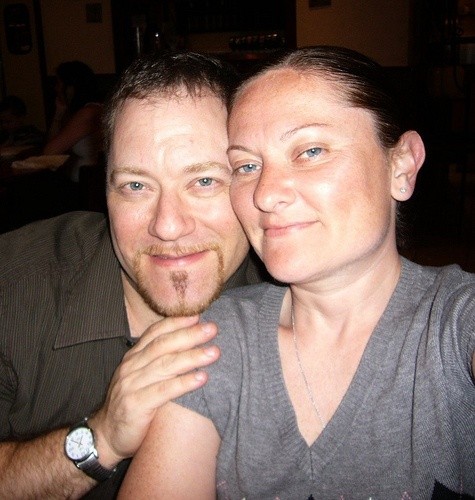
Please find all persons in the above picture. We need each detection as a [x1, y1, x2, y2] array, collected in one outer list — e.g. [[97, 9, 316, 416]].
[[114, 41, 474, 500], [2, 52, 273, 499], [0, 96, 47, 149], [41, 59, 112, 184]]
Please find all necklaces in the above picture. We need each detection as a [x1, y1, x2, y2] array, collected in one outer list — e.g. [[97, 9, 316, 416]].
[[287, 298, 331, 433]]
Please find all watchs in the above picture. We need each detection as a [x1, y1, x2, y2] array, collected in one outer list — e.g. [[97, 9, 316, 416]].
[[64, 411, 117, 484]]
